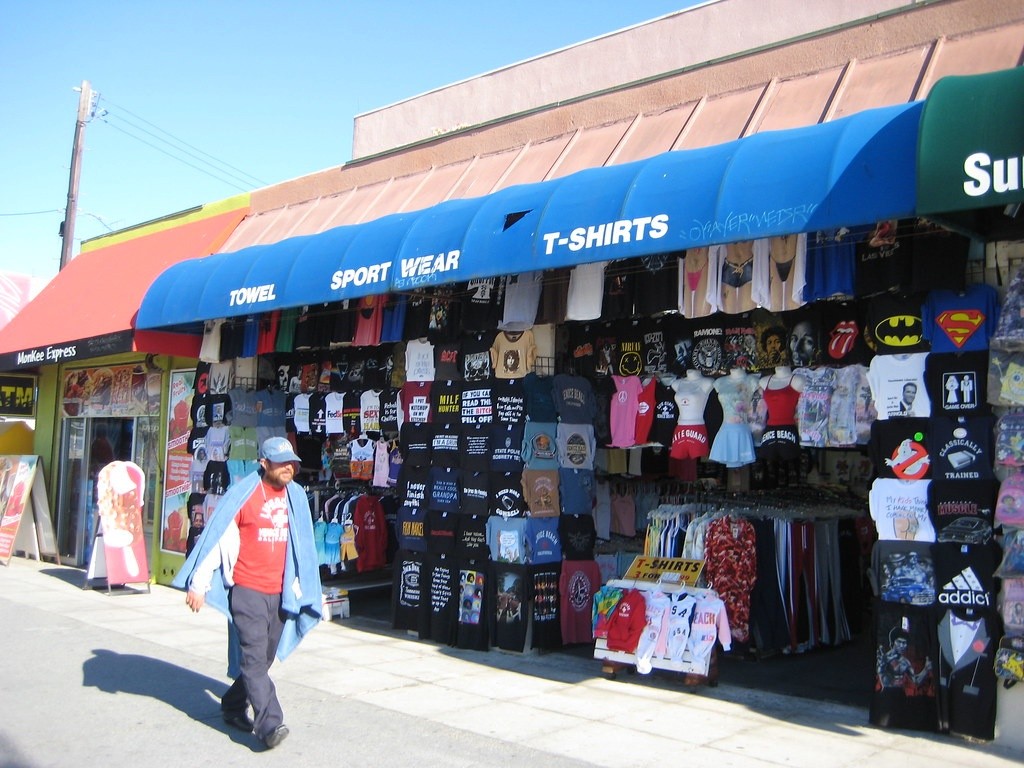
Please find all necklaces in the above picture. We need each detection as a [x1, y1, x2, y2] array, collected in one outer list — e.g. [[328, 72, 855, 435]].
[[259, 475, 288, 532]]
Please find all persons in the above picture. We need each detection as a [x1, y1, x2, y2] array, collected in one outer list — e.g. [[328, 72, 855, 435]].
[[682, 229, 824, 319], [900, 382, 919, 415], [670, 363, 806, 470], [185, 436, 332, 750], [192, 512, 204, 529], [876, 626, 932, 699]]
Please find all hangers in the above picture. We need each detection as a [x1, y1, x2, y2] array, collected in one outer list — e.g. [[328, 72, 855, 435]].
[[616, 477, 868, 525]]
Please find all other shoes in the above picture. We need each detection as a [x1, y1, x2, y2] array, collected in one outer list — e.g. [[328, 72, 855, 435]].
[[264, 722, 290, 748], [223, 709, 255, 731]]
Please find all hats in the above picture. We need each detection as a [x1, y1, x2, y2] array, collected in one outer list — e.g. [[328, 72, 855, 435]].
[[259, 436, 302, 463]]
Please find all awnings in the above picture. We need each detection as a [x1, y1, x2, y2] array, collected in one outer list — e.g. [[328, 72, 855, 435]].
[[133, 98, 932, 335], [0, 205, 251, 372], [916, 64, 1024, 245]]
